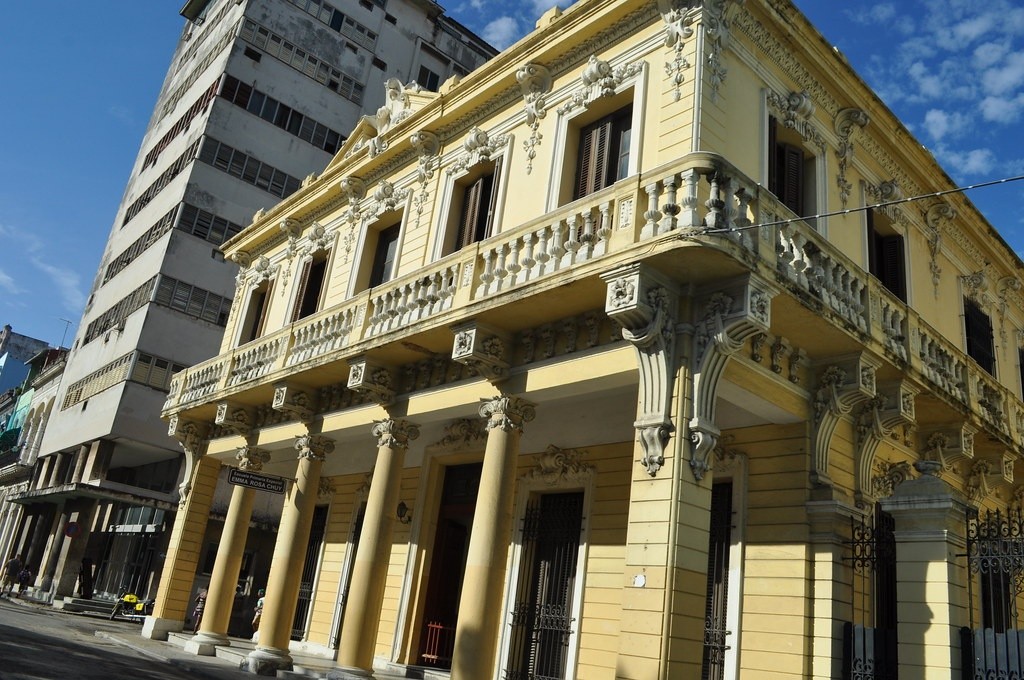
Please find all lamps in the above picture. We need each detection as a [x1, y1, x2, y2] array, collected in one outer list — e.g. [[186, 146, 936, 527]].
[[396, 501, 412, 525]]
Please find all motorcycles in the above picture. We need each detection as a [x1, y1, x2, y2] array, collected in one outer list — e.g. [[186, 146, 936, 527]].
[[110, 585, 154, 623]]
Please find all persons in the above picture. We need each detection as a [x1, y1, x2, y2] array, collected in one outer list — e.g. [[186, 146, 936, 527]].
[[251, 589, 265, 631], [15, 565, 33, 598], [0, 554, 23, 597], [193, 585, 210, 633]]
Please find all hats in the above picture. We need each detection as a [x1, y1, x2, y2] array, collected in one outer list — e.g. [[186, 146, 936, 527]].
[[259, 589, 265, 593]]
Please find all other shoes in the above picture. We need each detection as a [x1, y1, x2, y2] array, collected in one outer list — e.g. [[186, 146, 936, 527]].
[[15, 594, 18, 598], [0, 592, 3, 597], [7, 594, 12, 597]]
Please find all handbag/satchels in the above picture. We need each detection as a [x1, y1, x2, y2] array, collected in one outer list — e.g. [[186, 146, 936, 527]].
[[193, 611, 197, 616]]
